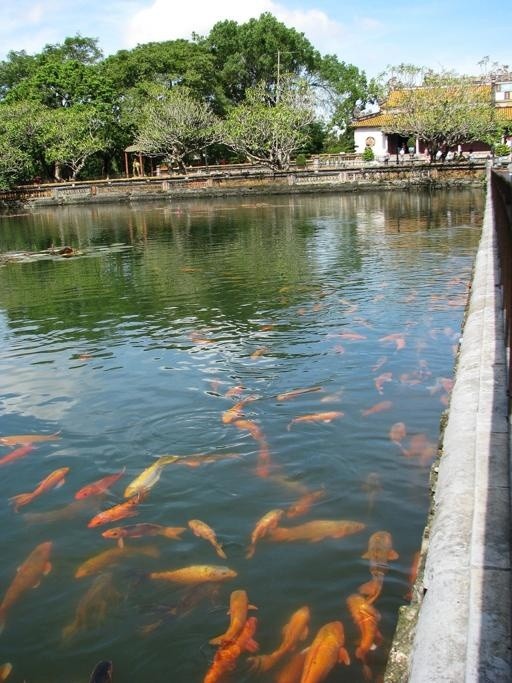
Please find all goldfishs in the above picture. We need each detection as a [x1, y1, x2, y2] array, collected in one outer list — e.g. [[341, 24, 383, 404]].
[[1, 265, 480, 682]]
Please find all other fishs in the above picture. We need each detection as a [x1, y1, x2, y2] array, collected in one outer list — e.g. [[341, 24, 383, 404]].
[[1, 265, 480, 682]]
